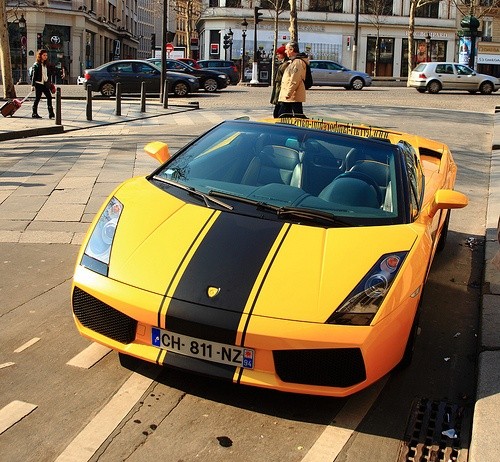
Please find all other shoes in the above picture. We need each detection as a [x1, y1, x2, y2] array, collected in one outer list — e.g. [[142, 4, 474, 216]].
[[32, 113, 42, 118], [49, 114, 56, 120]]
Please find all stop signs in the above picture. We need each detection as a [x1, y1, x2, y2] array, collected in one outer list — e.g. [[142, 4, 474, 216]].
[[167, 44, 174, 52]]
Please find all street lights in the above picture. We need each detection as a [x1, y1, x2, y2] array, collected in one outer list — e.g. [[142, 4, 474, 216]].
[[227, 30, 234, 60], [425, 36, 432, 62], [18, 17, 32, 86], [241, 20, 248, 82]]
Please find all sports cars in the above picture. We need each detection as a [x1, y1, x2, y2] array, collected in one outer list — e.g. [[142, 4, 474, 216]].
[[71, 115, 470, 396]]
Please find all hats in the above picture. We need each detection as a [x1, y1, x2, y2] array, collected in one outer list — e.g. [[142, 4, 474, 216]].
[[276, 46, 287, 57]]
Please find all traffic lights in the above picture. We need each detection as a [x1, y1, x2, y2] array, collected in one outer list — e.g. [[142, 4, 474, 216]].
[[223, 36, 230, 50], [254, 6, 263, 23], [166, 31, 176, 43]]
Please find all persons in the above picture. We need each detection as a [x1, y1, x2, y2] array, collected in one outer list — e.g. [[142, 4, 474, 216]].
[[31, 49, 63, 119], [270, 40, 309, 118], [415, 42, 431, 62], [303, 44, 313, 60], [245, 50, 250, 61], [257, 43, 266, 60], [461, 42, 470, 52]]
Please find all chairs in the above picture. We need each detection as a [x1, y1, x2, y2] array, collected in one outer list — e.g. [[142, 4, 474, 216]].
[[241, 132, 390, 208], [447, 66, 452, 71]]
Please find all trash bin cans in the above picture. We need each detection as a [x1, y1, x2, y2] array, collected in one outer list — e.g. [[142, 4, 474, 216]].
[[259, 62, 270, 82]]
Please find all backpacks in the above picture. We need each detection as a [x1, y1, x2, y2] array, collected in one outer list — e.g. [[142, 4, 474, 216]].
[[292, 57, 313, 90]]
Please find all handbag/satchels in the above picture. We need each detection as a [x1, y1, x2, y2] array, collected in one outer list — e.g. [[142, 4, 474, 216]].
[[50, 84, 56, 94]]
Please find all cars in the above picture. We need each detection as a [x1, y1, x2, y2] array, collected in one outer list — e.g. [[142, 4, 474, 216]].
[[83, 59, 198, 98], [147, 59, 229, 93], [309, 60, 372, 91], [407, 64, 500, 95], [186, 60, 240, 86], [175, 58, 201, 69]]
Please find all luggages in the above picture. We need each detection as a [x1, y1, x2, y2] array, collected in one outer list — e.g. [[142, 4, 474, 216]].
[[0, 91, 34, 117]]
